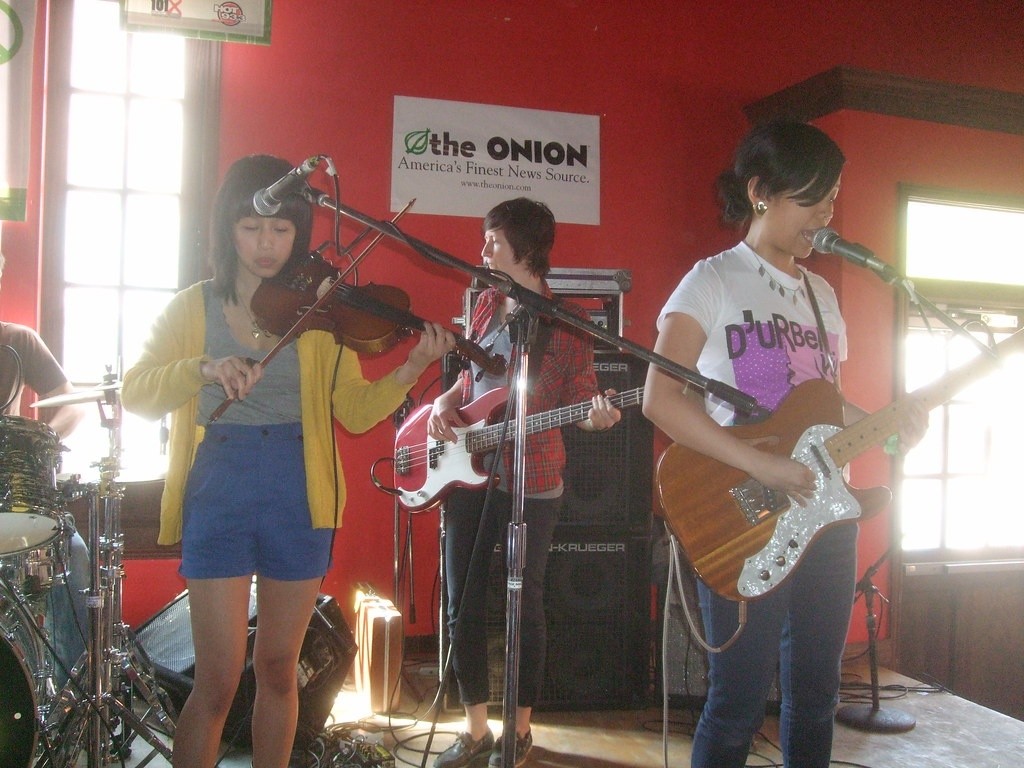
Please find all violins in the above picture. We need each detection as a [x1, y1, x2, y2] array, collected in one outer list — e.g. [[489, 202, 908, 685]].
[[249, 251, 508, 383]]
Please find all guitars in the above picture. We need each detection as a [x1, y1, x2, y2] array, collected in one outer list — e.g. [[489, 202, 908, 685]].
[[393, 386, 644, 514], [654, 329, 1024, 602]]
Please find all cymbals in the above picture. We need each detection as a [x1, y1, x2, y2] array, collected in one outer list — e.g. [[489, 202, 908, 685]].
[[30, 383, 127, 409]]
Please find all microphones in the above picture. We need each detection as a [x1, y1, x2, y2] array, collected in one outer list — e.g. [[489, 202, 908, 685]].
[[811, 227, 906, 285], [254, 155, 327, 216]]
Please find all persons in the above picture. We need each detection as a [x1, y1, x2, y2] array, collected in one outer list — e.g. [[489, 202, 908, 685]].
[[0, 253, 131, 761], [427, 198, 621, 768], [120, 155, 456, 768], [642, 119, 929, 768]]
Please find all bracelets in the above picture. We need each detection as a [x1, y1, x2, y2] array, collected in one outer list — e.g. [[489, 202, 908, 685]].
[[883, 434, 899, 457]]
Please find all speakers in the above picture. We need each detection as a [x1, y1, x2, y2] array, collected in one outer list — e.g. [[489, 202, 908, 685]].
[[125, 573, 358, 751], [654, 562, 783, 716], [437, 532, 653, 715], [443, 346, 666, 536]]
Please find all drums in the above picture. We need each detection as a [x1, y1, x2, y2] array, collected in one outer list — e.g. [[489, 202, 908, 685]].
[[0, 582, 49, 768], [0, 511, 76, 597], [0, 415, 65, 558]]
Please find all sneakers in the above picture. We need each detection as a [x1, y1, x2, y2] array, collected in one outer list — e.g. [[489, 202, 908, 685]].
[[488, 728, 532, 768], [433, 729, 494, 768]]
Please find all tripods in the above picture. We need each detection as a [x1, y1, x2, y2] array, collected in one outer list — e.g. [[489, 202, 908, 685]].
[[32, 398, 181, 768]]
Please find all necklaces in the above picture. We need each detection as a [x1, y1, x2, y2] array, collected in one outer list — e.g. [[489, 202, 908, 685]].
[[744, 238, 807, 304], [235, 287, 262, 340]]
